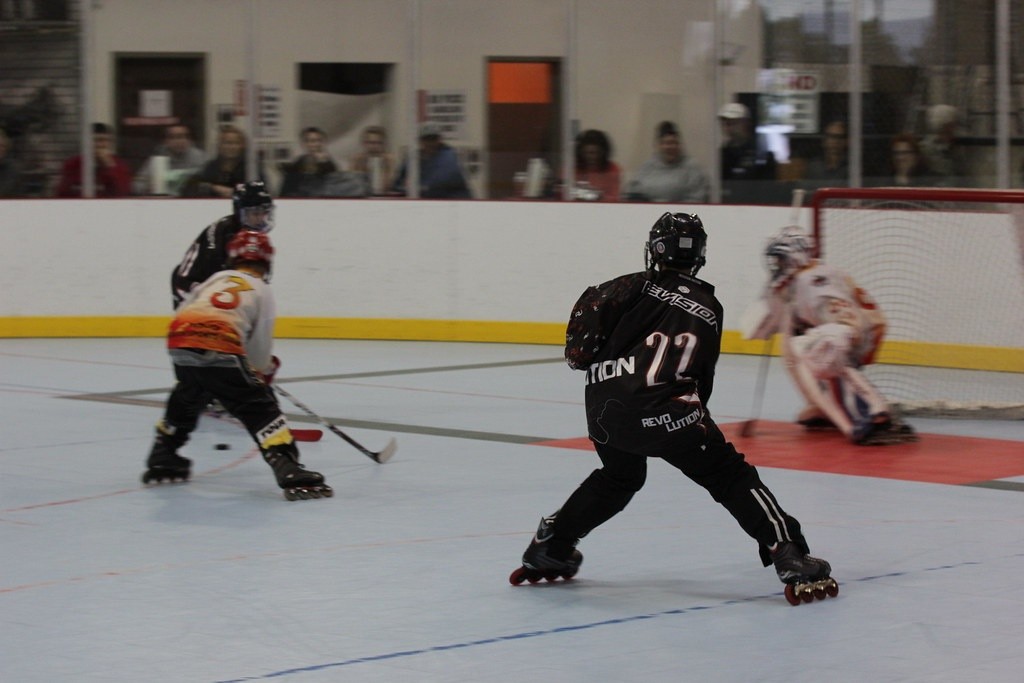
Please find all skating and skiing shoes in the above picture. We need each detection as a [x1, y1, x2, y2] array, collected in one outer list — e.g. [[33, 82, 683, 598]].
[[142, 436, 191, 483], [509, 517, 582, 587], [260, 446, 333, 501], [763, 540, 839, 607]]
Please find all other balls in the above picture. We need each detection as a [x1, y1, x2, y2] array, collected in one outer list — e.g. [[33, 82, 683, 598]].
[[216, 444, 227, 450]]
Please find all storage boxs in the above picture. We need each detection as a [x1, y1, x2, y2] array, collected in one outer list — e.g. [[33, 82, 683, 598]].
[[759, 94, 822, 134]]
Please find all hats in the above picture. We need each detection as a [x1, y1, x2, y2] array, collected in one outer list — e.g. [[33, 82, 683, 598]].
[[417, 124, 440, 138], [717, 103, 750, 119], [658, 121, 679, 137]]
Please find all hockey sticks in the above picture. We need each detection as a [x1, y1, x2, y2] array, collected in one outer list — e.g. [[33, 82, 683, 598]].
[[739, 189, 806, 438], [266, 378, 398, 466]]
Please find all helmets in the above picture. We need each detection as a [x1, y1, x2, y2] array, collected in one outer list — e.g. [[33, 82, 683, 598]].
[[225, 229, 274, 261], [765, 226, 816, 289], [644, 211, 708, 277], [232, 181, 274, 233]]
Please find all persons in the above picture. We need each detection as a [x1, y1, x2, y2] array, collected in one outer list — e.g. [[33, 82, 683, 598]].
[[741, 225, 919, 445], [146, 229, 326, 488], [171, 180, 275, 416], [523, 212, 831, 585], [509, 103, 971, 213], [55, 121, 474, 201]]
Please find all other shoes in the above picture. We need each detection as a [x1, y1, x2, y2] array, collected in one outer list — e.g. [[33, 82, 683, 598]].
[[796, 407, 838, 431], [853, 412, 917, 445]]
